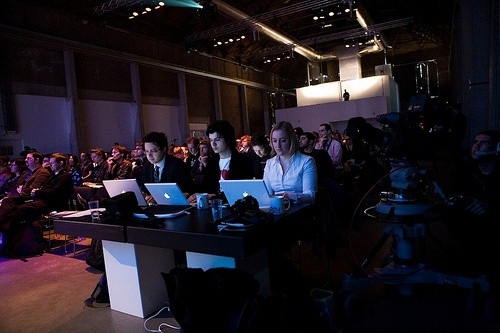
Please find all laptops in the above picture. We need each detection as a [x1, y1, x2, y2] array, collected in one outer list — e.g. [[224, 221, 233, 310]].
[[143, 182, 210, 207], [102, 179, 155, 207], [219, 178, 290, 208]]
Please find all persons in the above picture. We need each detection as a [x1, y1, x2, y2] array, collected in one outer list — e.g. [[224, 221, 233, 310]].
[[0, 141, 147, 257], [343, 90, 349, 101], [263, 121, 318, 204], [183, 120, 254, 206], [170, 123, 352, 186], [343, 117, 383, 189], [453, 128, 500, 253], [135, 131, 195, 203]]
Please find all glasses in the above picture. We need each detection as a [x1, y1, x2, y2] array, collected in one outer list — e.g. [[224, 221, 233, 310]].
[[143, 148, 161, 154]]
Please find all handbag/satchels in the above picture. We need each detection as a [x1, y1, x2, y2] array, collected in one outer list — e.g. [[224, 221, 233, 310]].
[[83, 271, 108, 306]]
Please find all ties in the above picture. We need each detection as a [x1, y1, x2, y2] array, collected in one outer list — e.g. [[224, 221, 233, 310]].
[[154, 166, 160, 182]]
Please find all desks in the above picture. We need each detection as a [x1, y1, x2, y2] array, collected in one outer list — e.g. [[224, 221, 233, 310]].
[[54, 205, 307, 320]]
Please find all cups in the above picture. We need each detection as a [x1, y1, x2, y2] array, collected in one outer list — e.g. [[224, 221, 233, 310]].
[[88, 201, 99, 220], [196, 193, 209, 210], [209, 199, 223, 221], [269, 195, 290, 215]]
